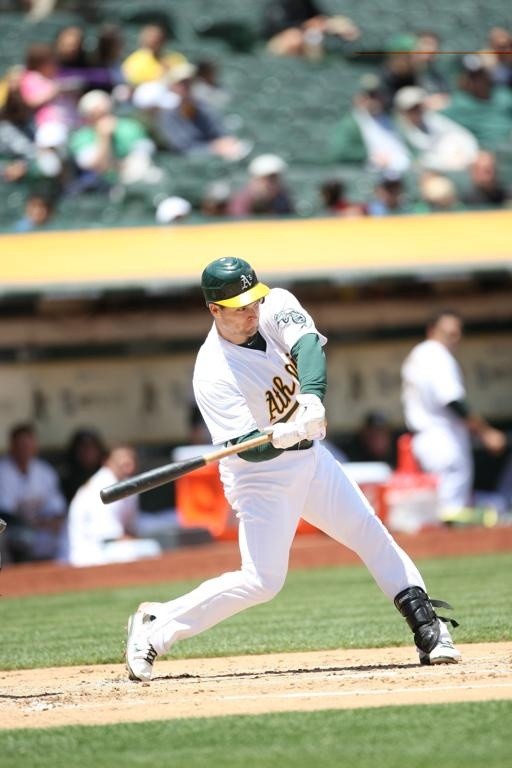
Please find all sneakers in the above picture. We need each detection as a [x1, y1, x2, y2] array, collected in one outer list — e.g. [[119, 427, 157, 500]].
[[123, 612, 159, 685], [416, 621, 464, 665]]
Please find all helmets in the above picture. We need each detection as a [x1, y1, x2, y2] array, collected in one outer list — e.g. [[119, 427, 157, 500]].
[[199, 257, 271, 310]]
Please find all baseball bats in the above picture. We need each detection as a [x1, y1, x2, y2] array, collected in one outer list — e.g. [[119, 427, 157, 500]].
[[100, 433, 271, 505]]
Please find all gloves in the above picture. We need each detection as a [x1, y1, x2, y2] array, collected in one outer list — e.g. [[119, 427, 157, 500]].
[[262, 393, 328, 450]]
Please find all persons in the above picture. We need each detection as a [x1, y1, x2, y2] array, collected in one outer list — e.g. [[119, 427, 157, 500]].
[[0, 421, 66, 561], [120, 253, 463, 681], [67, 444, 163, 568], [262, 1, 361, 65], [346, 410, 398, 472], [399, 311, 508, 525], [184, 404, 213, 444], [58, 427, 110, 507], [0, 19, 298, 235], [320, 28, 512, 215]]
